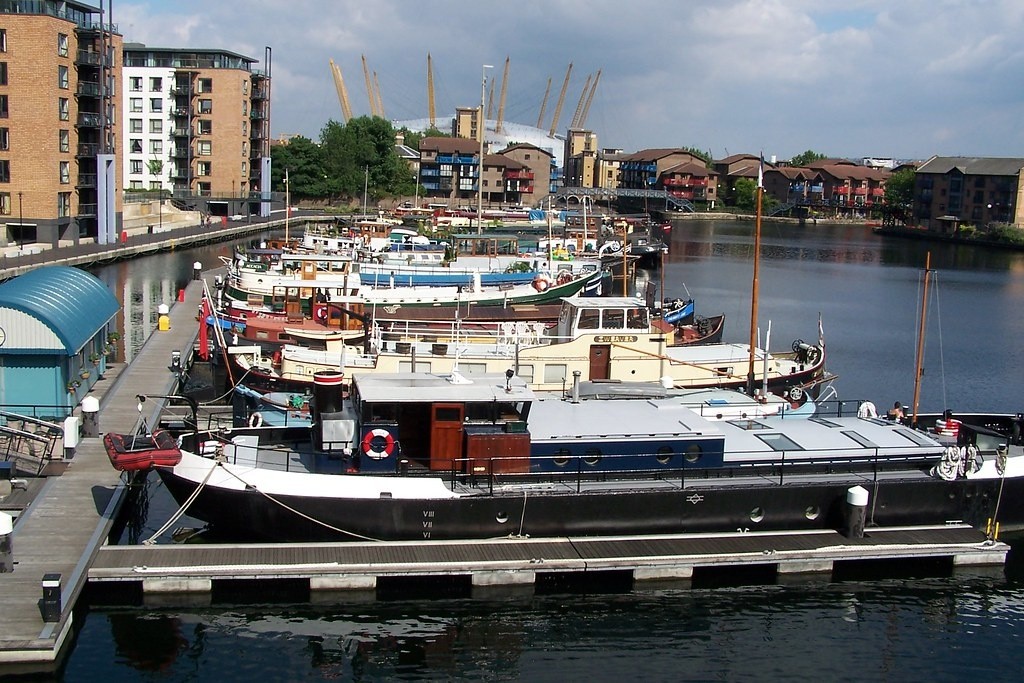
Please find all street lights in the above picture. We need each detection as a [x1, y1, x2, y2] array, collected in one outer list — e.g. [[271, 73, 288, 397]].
[[477, 64, 496, 235]]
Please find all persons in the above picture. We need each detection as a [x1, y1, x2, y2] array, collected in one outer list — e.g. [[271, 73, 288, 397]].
[[891, 401, 904, 422]]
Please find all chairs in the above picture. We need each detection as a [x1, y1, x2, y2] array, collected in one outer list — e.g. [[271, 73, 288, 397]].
[[887, 411, 896, 422], [501, 322, 549, 345], [900, 417, 911, 428]]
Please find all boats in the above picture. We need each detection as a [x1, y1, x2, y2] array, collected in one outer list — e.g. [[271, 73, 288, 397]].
[[82, 201, 1024, 572]]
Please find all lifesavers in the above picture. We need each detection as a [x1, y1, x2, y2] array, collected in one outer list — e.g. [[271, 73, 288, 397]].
[[249, 412, 262, 428], [317, 307, 328, 319], [363, 428, 394, 460], [199, 440, 225, 453], [557, 272, 574, 285], [935, 419, 963, 437], [537, 279, 549, 290]]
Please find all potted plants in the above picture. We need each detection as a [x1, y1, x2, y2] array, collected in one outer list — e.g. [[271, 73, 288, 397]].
[[107, 331, 120, 344], [80, 368, 91, 379], [89, 352, 101, 365], [100, 342, 111, 355], [68, 379, 81, 393]]
